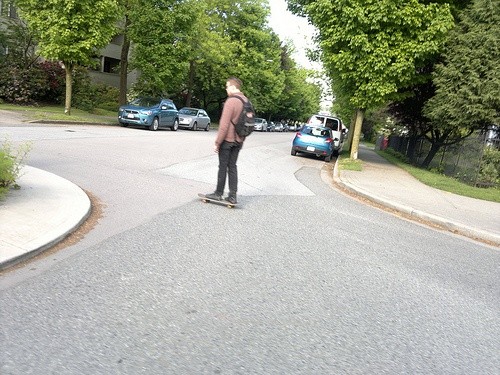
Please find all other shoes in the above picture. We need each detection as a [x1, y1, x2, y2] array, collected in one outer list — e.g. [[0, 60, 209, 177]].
[[205, 192, 221, 201], [225, 196, 237, 204]]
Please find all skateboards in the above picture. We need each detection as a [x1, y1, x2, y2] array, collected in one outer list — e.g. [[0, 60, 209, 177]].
[[197, 193, 242, 209]]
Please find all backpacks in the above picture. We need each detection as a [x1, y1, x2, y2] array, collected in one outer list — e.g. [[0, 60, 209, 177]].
[[225, 95, 256, 138]]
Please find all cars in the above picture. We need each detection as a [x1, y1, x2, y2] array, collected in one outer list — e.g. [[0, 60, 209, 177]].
[[253, 117, 268, 132], [177, 106, 211, 132], [291, 123, 339, 162], [266, 120, 306, 133], [117, 97, 179, 131]]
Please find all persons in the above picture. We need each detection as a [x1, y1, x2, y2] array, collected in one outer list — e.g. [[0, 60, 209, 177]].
[[205, 78, 250, 204]]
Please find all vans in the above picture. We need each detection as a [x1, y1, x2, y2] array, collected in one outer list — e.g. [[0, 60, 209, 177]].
[[307, 114, 344, 157]]
